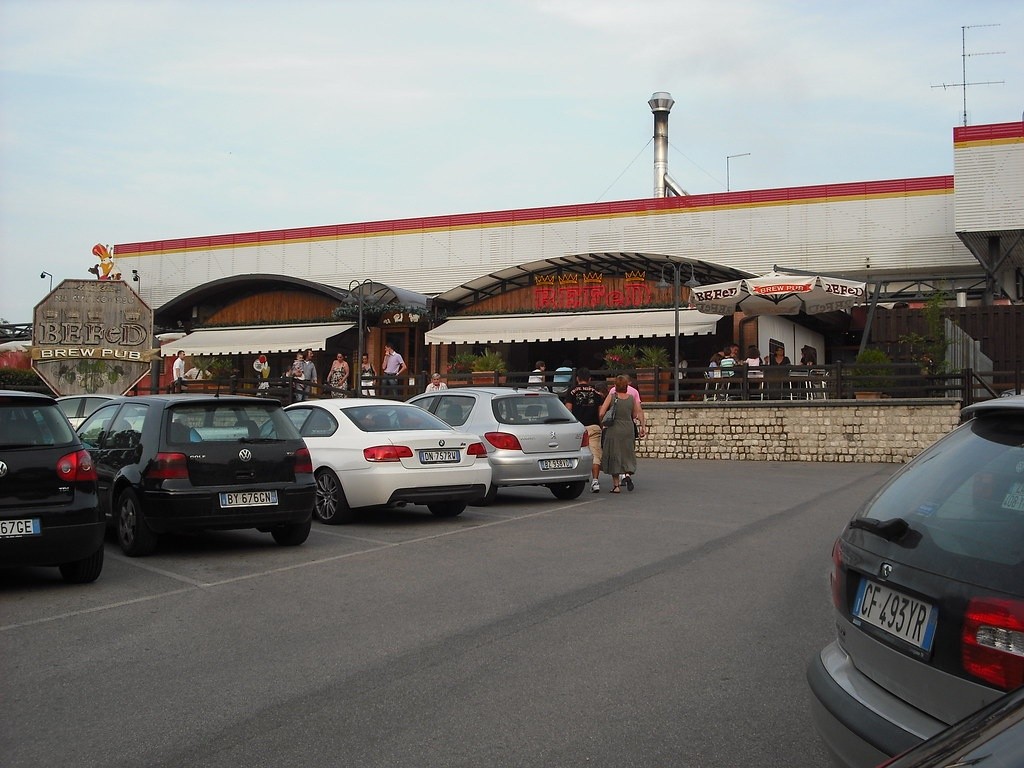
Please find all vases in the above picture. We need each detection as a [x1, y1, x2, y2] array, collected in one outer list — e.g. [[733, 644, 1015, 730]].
[[447, 381, 473, 384], [606, 377, 637, 394]]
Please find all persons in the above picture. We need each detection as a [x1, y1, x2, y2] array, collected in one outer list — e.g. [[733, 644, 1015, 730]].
[[527, 361, 549, 392], [609, 376, 645, 486], [599, 375, 638, 493], [565, 368, 605, 493], [553, 360, 572, 394], [380, 343, 407, 397], [361, 353, 376, 396], [293, 351, 305, 388], [185, 366, 212, 380], [426, 373, 448, 392], [173, 350, 185, 382], [327, 353, 349, 398], [284, 349, 318, 401], [708, 343, 816, 402]]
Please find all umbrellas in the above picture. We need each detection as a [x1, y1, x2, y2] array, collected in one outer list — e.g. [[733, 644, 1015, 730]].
[[690, 272, 867, 314]]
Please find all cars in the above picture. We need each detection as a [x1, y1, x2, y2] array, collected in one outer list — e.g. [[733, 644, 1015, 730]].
[[257, 398, 492, 524], [0, 390, 107, 583], [808, 394, 1023, 768], [75, 393, 317, 556], [386, 386, 594, 507], [34, 394, 188, 443]]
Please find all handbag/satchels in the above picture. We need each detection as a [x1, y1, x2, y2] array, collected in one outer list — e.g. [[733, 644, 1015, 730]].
[[370, 363, 378, 383], [600, 393, 617, 426]]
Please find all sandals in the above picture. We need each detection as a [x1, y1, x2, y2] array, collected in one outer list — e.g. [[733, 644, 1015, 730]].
[[626, 473, 634, 491], [608, 485, 620, 493]]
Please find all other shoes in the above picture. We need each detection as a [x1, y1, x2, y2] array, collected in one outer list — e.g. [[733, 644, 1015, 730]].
[[619, 474, 626, 486], [590, 481, 600, 493]]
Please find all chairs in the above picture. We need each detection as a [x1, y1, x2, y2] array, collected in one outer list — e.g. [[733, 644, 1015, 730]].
[[102, 419, 135, 449], [444, 404, 464, 426], [371, 413, 391, 428], [702, 369, 831, 401], [310, 415, 332, 435], [0, 419, 40, 445]]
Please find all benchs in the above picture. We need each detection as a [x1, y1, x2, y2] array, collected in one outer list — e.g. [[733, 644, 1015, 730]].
[[358, 418, 424, 429], [171, 421, 261, 442]]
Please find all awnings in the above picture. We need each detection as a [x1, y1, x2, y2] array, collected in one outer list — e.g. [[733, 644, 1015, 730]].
[[424, 306, 733, 344], [160, 321, 359, 356]]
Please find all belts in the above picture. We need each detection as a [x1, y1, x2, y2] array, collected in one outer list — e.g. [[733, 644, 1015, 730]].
[[384, 372, 396, 376]]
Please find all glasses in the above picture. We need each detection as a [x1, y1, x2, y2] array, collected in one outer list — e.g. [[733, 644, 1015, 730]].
[[542, 366, 546, 368]]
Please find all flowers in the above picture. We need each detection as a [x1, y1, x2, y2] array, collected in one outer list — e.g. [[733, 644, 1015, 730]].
[[603, 344, 637, 378], [444, 351, 478, 381]]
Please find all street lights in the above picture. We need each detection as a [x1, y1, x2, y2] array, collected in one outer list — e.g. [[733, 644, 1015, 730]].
[[343, 279, 377, 393], [131, 270, 140, 295], [40, 271, 53, 292], [655, 261, 701, 401]]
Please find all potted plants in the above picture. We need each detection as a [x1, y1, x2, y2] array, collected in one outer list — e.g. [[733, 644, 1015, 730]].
[[854, 349, 892, 399], [635, 346, 672, 402], [469, 347, 507, 384]]
